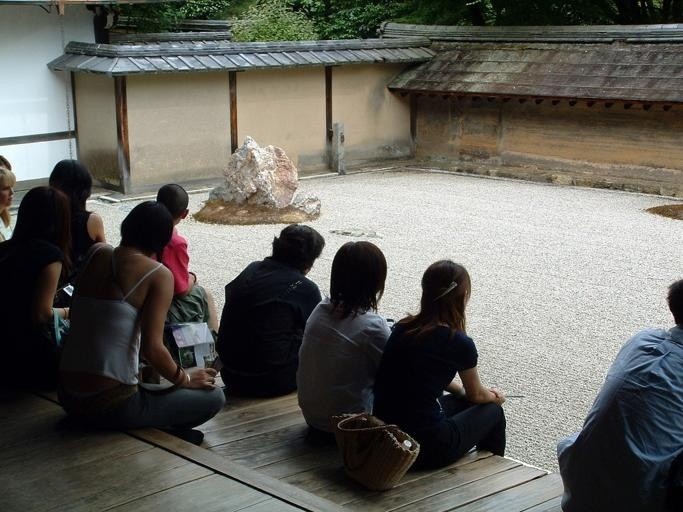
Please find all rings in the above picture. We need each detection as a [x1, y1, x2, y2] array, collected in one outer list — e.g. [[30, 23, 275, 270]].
[[213, 377, 216, 383]]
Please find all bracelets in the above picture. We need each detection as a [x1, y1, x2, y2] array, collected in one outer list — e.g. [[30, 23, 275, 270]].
[[63, 308, 68, 321], [174, 365, 181, 382]]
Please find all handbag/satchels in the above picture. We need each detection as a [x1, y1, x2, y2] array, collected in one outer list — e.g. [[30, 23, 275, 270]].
[[329, 413, 420, 490], [42, 316, 69, 346]]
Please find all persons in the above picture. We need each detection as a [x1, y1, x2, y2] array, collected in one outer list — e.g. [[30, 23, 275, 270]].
[[559, 277, 682, 512], [150, 184, 218, 339], [295, 242, 394, 443], [55, 202, 227, 446], [372, 261, 506, 473], [49, 159, 105, 284], [0, 170, 17, 242], [1, 154, 13, 170], [0, 185, 70, 399], [215, 224, 325, 398]]
